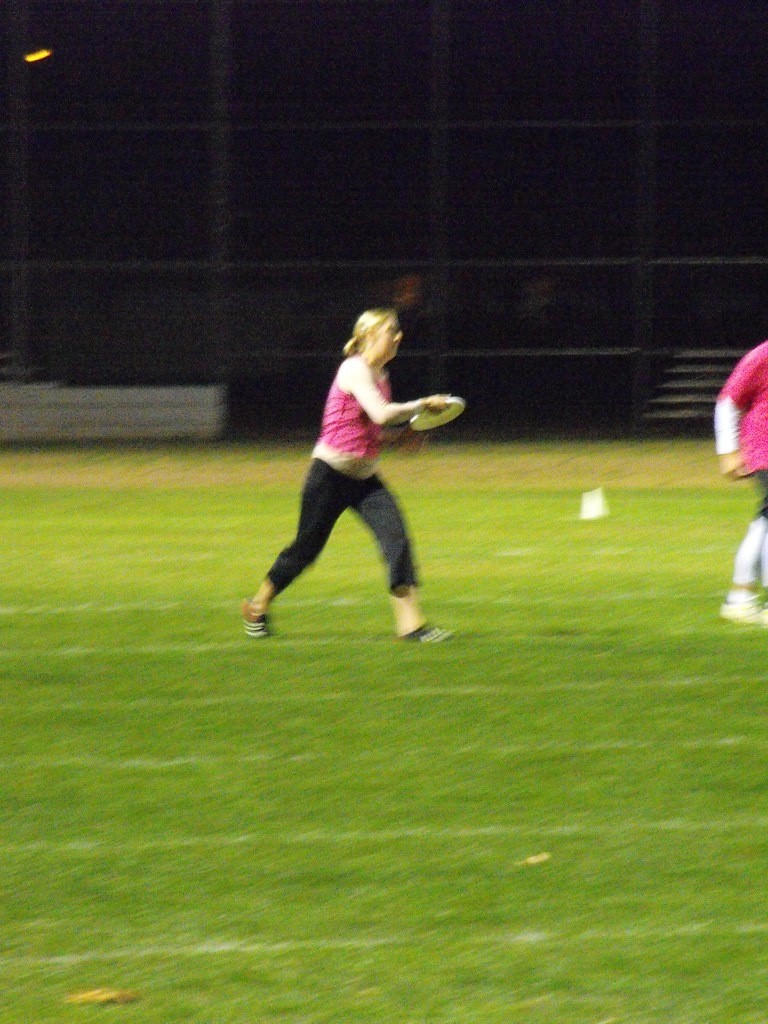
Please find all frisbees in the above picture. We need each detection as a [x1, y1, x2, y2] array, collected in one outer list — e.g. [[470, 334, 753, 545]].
[[410, 396, 466, 431]]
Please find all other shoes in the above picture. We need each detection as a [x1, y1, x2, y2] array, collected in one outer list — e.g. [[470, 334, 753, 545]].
[[242, 595, 269, 640], [720, 594, 765, 625], [401, 620, 453, 645]]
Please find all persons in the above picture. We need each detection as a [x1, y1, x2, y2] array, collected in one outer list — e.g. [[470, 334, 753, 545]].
[[716, 341, 767, 624], [241, 307, 456, 643]]
